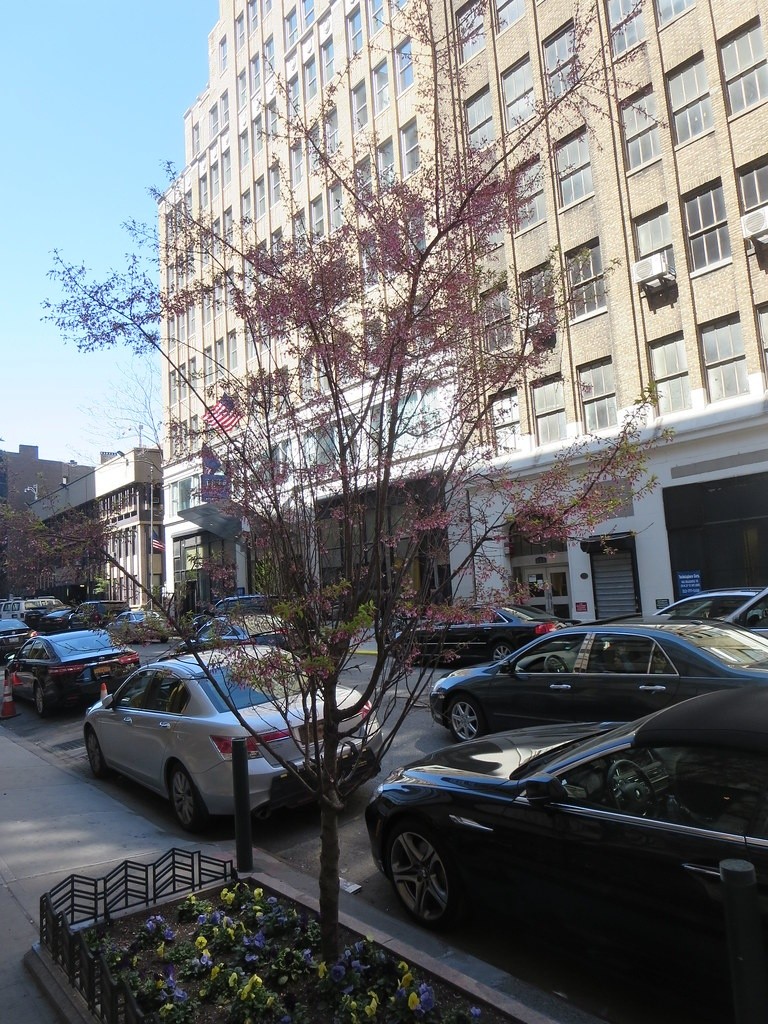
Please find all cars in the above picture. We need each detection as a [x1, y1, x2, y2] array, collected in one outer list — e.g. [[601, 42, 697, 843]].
[[652, 587, 766, 626], [721, 588, 768, 638], [39, 609, 74, 633], [4, 628, 141, 717], [384, 604, 583, 668], [0, 619, 38, 664], [174, 614, 316, 658], [430, 612, 767, 742], [82, 644, 384, 830], [360, 683, 768, 1024], [106, 611, 168, 643]]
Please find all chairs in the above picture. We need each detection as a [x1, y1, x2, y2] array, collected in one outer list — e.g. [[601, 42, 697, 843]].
[[164, 684, 187, 714]]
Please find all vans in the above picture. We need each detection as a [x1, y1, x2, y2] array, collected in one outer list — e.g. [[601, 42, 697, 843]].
[[0, 595, 64, 622]]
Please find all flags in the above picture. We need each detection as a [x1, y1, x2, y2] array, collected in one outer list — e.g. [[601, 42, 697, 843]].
[[203, 394, 245, 433], [153, 529, 165, 551], [200, 441, 222, 475]]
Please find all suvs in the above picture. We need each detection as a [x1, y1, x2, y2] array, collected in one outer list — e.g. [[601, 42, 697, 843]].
[[67, 601, 131, 630], [191, 594, 296, 634]]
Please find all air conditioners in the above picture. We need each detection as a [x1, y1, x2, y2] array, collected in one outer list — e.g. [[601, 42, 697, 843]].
[[520, 305, 559, 337], [631, 252, 667, 286], [741, 206, 768, 241]]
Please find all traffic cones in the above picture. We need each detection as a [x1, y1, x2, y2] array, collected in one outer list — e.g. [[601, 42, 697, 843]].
[[0, 667, 21, 719], [99, 683, 110, 700]]
